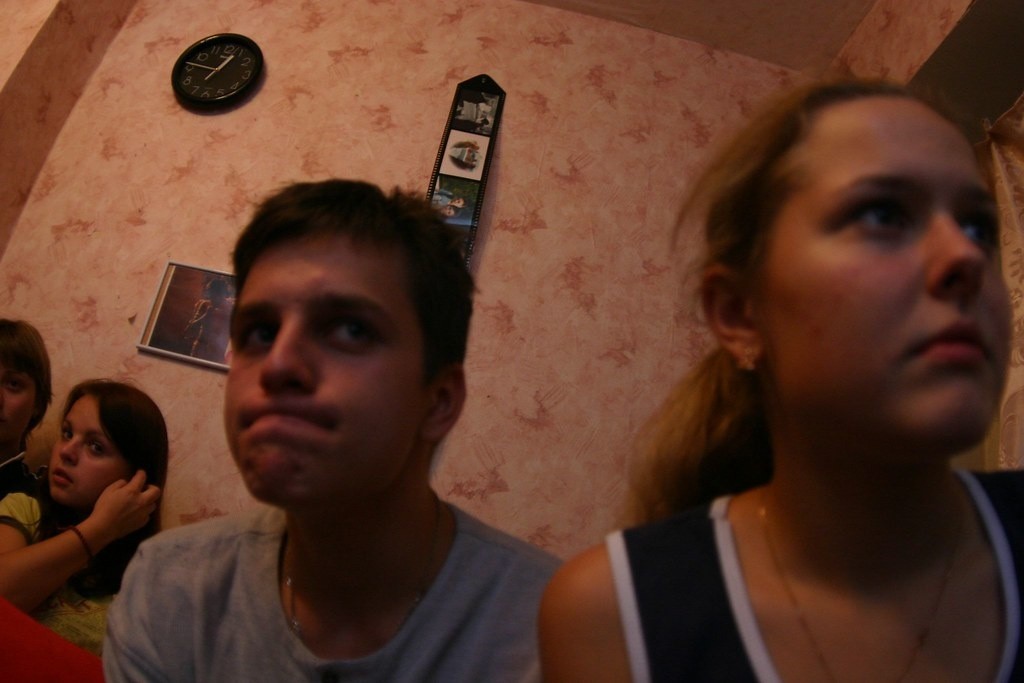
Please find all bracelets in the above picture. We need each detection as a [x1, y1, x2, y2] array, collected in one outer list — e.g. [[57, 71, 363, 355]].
[[58, 524, 93, 569]]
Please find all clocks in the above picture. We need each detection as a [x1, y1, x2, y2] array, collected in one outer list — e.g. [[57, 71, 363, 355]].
[[172, 33, 264, 109]]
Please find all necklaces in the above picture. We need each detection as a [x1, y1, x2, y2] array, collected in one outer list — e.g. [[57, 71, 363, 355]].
[[755, 492, 956, 683]]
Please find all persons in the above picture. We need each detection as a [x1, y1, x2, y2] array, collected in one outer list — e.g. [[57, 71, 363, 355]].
[[99, 178, 571, 683], [0, 319, 168, 683], [536, 66, 1024, 683]]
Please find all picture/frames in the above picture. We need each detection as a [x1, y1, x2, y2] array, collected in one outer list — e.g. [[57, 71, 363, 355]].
[[136, 261, 235, 371]]
